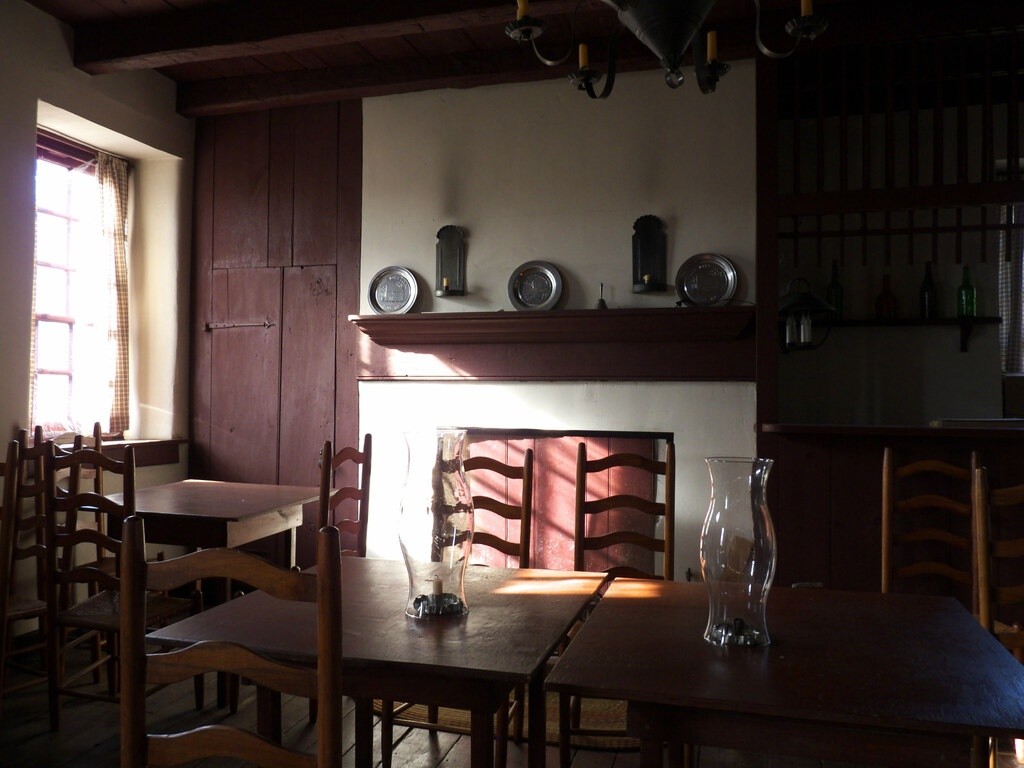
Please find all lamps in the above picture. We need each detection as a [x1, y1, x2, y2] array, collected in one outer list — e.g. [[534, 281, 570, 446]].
[[777, 279, 840, 358], [505, 0, 829, 100]]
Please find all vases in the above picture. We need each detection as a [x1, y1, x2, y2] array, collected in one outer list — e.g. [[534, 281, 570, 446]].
[[701, 456, 779, 649], [394, 428, 476, 622]]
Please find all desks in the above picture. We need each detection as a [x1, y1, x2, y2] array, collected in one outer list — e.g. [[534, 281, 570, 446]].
[[543, 556, 1024, 768], [142, 555, 609, 768], [78, 477, 339, 709], [762, 418, 1024, 633]]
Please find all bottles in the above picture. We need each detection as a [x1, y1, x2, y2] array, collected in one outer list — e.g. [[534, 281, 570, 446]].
[[919, 261, 938, 320], [875, 274, 896, 318], [395, 428, 475, 622], [825, 259, 843, 319], [699, 456, 778, 649], [956, 265, 977, 318]]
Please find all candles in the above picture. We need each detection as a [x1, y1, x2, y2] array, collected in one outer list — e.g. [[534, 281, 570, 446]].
[[444, 276, 449, 287], [801, 0, 813, 16], [579, 42, 588, 69], [516, 0, 529, 21], [707, 30, 716, 65], [645, 274, 650, 284]]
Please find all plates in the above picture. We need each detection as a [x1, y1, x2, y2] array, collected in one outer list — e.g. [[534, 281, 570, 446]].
[[368, 266, 419, 315], [675, 253, 738, 307], [508, 261, 562, 311]]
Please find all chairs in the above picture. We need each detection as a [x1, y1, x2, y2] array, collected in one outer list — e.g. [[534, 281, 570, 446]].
[[0, 424, 1024, 768], [316, 433, 371, 560]]
